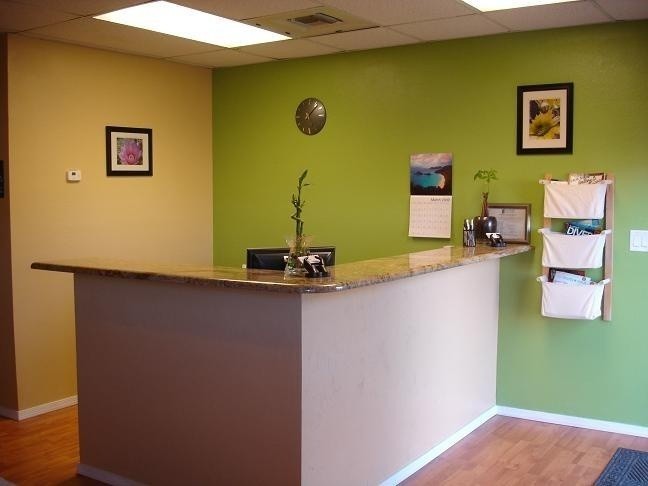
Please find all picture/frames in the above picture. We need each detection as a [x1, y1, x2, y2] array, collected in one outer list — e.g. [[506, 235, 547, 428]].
[[483, 203, 532, 245], [106, 126, 153, 177], [516, 82, 574, 155]]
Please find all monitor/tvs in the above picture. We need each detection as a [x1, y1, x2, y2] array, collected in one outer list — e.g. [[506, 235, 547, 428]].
[[247, 246, 336, 270]]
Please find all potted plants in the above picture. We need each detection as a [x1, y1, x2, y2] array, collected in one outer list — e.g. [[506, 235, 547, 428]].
[[282, 169, 313, 279], [473, 168, 498, 239]]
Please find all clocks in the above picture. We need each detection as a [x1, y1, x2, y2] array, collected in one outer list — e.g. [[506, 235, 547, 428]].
[[295, 98, 327, 135]]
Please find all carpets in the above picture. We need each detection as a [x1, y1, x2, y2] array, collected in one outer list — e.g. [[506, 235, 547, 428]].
[[593, 447, 648, 486]]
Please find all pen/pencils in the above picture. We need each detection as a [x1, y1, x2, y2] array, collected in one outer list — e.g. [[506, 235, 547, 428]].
[[463, 219, 475, 247]]
